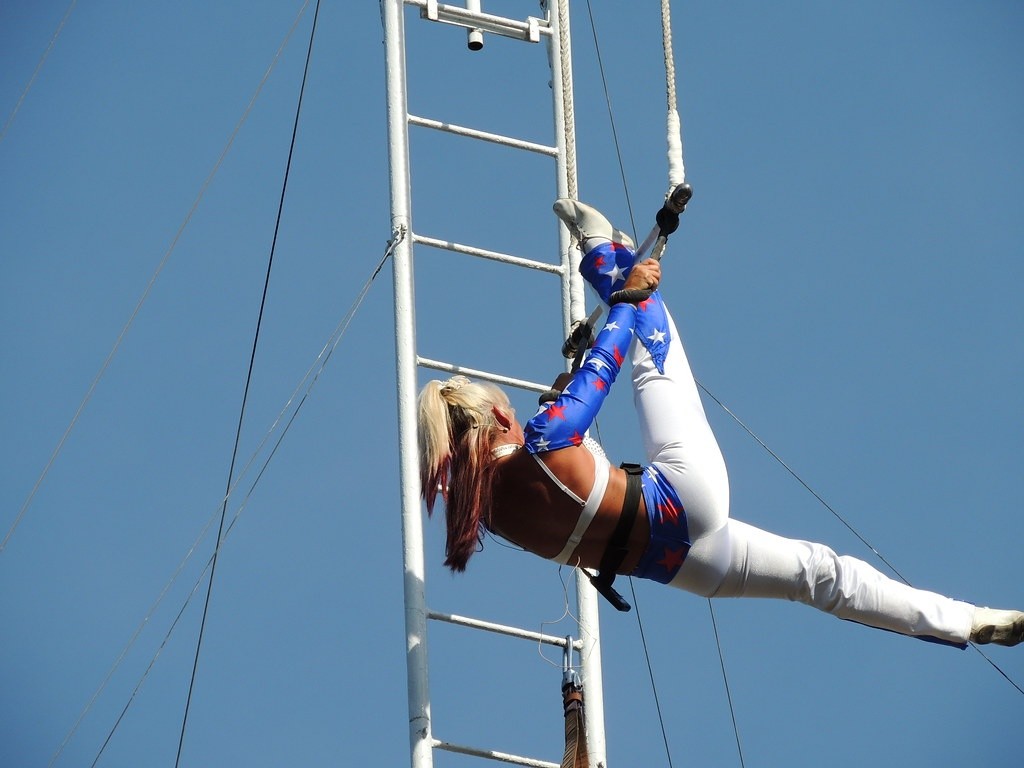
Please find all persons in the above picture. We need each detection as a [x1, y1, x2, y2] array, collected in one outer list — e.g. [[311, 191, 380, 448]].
[[417, 198, 1024, 647]]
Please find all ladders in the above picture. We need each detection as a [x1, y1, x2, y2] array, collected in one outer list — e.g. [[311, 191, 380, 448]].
[[378, 0, 606, 768]]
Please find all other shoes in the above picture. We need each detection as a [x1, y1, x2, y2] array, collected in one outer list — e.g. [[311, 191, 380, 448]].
[[969, 607, 1024, 647], [553, 198, 634, 255]]
